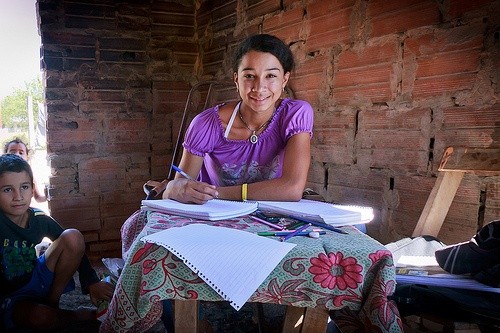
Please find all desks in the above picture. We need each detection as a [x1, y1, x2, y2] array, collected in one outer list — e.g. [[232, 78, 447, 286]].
[[100, 211, 402, 333]]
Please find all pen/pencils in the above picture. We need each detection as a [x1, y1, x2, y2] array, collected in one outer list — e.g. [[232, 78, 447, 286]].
[[249, 210, 349, 242]]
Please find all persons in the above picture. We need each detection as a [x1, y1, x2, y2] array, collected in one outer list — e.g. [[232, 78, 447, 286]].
[[0, 153, 115, 333], [2, 137, 49, 203], [161, 34, 313, 333]]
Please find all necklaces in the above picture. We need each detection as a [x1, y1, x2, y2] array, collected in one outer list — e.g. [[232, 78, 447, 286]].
[[238, 101, 270, 144]]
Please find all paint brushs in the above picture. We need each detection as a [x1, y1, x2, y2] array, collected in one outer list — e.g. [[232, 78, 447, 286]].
[[171, 164, 219, 199]]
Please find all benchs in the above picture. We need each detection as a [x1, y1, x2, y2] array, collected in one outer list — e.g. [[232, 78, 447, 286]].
[[396, 273, 500, 333]]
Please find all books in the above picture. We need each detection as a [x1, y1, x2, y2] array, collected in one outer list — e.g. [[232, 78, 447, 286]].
[[141, 195, 374, 228]]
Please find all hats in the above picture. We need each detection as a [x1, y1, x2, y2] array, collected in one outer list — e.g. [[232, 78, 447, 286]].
[[435, 220, 500, 274]]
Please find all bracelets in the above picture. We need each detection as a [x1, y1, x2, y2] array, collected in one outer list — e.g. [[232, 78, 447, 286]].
[[241, 183, 247, 201]]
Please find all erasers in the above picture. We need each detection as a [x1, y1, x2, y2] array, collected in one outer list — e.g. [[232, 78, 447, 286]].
[[309, 232, 319, 239]]
